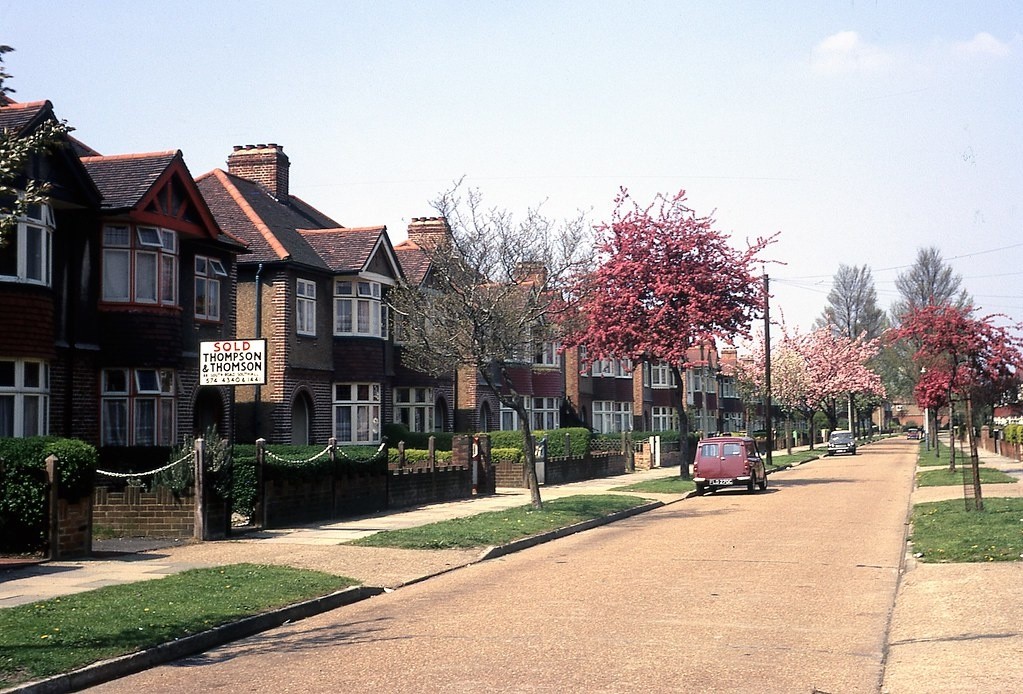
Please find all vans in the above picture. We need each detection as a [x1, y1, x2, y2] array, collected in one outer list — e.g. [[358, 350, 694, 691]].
[[693, 437, 767, 497]]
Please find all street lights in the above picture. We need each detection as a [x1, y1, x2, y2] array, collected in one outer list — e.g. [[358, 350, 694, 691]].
[[920, 366, 929, 451]]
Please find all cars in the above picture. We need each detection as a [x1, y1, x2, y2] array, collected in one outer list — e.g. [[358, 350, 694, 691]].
[[828, 431, 856, 456], [907, 429, 919, 440]]
